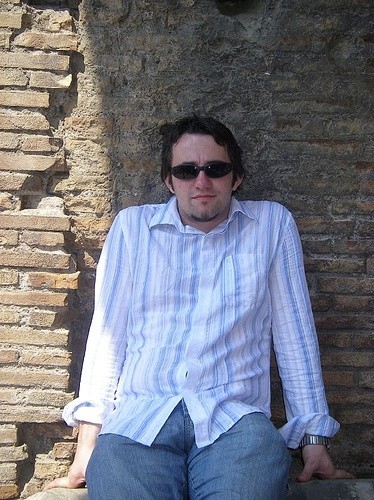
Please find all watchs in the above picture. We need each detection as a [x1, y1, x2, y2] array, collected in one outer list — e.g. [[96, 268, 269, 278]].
[[298, 434, 330, 450]]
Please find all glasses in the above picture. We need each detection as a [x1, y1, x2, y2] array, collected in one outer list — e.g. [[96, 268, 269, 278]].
[[168, 161, 234, 180]]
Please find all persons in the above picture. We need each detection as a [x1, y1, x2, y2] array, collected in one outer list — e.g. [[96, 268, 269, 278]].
[[41, 119, 357, 500]]
